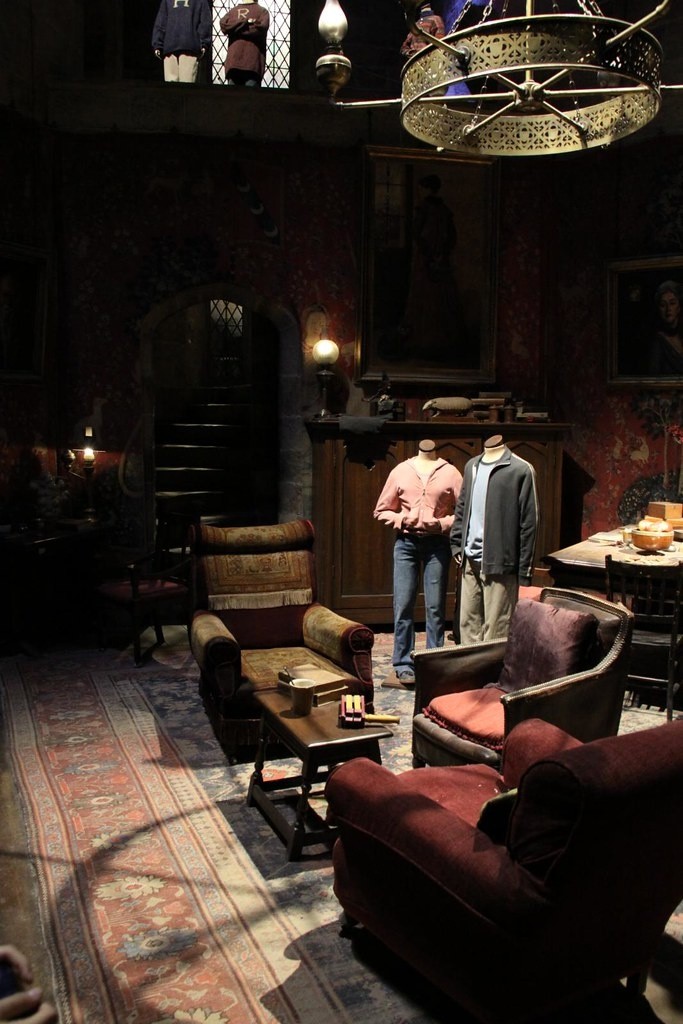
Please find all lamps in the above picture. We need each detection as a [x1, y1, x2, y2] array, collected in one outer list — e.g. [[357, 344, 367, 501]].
[[70, 438, 107, 516], [311, 339, 340, 418], [313, 0, 683, 158]]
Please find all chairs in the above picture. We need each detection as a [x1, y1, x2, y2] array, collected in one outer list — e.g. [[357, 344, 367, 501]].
[[602, 554, 682, 723], [412, 585, 636, 772], [89, 514, 194, 666]]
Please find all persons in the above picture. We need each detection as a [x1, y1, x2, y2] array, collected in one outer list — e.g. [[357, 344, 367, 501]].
[[374, 439, 464, 684], [399, 3, 451, 97], [449, 435, 541, 645], [219, 0, 269, 89], [151, 0, 213, 83]]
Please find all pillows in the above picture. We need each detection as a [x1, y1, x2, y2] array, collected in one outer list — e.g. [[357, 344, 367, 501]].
[[476, 787, 517, 839], [496, 601, 598, 695]]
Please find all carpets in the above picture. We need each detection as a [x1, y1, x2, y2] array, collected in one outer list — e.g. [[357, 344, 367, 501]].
[[2, 629, 683, 1024]]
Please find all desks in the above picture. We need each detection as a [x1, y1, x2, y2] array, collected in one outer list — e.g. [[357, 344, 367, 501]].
[[544, 522, 683, 669]]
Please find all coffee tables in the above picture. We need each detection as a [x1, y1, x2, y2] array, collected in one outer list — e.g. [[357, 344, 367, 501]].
[[245, 687, 392, 863]]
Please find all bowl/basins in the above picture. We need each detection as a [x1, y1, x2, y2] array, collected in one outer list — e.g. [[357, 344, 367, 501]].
[[630, 529, 674, 555]]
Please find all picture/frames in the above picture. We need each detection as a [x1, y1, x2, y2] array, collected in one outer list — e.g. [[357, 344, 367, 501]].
[[353, 144, 500, 386], [602, 252, 683, 392], [0, 238, 53, 388]]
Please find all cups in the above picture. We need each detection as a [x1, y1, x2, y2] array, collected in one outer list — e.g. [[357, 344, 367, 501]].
[[289, 679, 317, 717], [489, 404, 518, 423]]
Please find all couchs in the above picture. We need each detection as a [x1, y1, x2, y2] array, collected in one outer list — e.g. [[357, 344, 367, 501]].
[[184, 520, 377, 766], [320, 716, 683, 1023]]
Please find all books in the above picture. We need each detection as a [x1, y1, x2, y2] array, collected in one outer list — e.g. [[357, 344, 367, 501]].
[[278, 663, 350, 708]]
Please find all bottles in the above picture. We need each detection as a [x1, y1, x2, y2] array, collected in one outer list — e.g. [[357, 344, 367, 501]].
[[636, 511, 644, 525]]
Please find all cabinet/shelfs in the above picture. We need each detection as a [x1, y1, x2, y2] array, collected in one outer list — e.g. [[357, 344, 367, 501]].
[[0, 528, 80, 647]]
